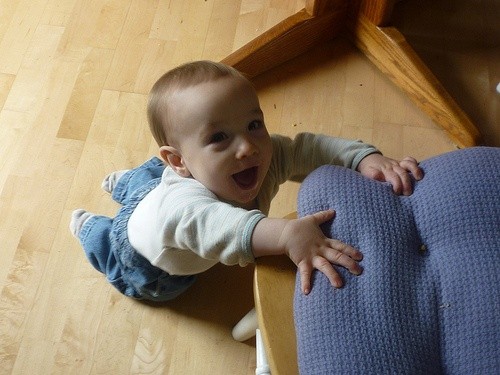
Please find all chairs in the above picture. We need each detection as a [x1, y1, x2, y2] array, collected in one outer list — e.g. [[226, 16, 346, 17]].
[[231, 212, 301, 375]]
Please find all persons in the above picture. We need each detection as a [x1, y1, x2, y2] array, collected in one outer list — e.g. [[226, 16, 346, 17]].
[[69, 61, 423, 302]]
